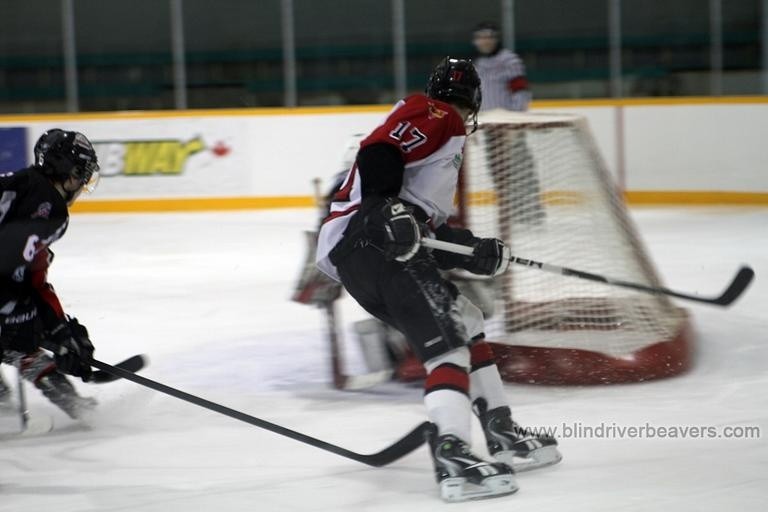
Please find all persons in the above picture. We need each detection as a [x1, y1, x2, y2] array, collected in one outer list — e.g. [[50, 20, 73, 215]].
[[308, 54, 560, 504], [0, 127, 102, 435], [449, 20, 533, 239], [288, 124, 498, 372]]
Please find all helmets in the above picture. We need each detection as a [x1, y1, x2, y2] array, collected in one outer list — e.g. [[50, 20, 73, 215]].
[[424, 56, 481, 111], [33, 128, 98, 195]]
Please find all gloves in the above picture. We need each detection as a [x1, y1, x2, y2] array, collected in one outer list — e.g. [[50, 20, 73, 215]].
[[49, 317, 94, 382], [431, 223, 510, 281], [0, 305, 43, 353], [373, 195, 420, 263]]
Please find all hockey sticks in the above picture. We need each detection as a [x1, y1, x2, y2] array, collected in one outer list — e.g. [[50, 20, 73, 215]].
[[80, 356, 437, 469]]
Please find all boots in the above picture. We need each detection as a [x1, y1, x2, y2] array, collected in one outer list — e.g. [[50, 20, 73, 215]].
[[33, 367, 97, 419], [423, 423, 513, 484], [471, 397, 557, 457]]
